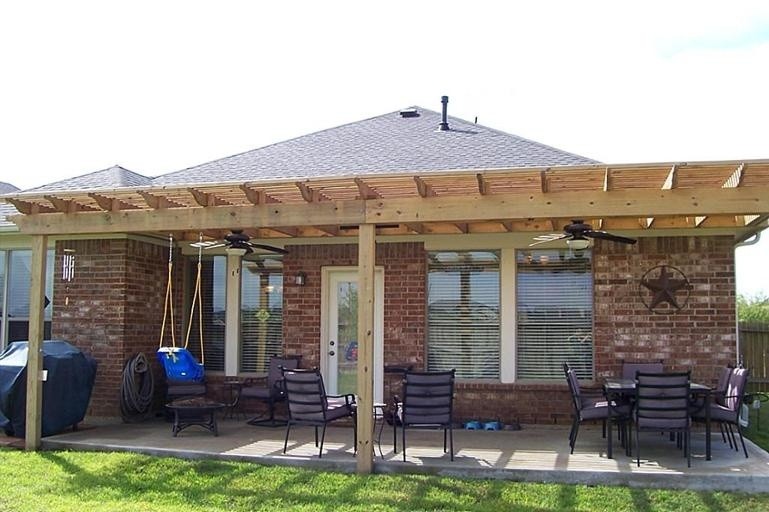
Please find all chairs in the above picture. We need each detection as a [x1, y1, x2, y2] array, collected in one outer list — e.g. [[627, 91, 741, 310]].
[[561, 356, 751, 468], [234, 352, 304, 429], [164, 379, 220, 437], [384, 367, 457, 464], [279, 365, 360, 461]]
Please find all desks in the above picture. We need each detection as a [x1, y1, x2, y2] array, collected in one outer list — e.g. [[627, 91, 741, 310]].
[[220, 378, 248, 424], [164, 398, 229, 439], [349, 401, 388, 460]]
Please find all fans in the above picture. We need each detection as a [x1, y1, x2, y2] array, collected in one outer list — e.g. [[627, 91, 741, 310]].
[[194, 227, 292, 258], [525, 218, 638, 250]]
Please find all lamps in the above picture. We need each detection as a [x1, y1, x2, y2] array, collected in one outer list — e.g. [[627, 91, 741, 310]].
[[294, 270, 305, 285], [566, 233, 590, 251], [223, 243, 248, 258]]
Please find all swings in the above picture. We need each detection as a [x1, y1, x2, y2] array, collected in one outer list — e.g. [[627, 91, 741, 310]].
[[156, 232, 205, 382]]
